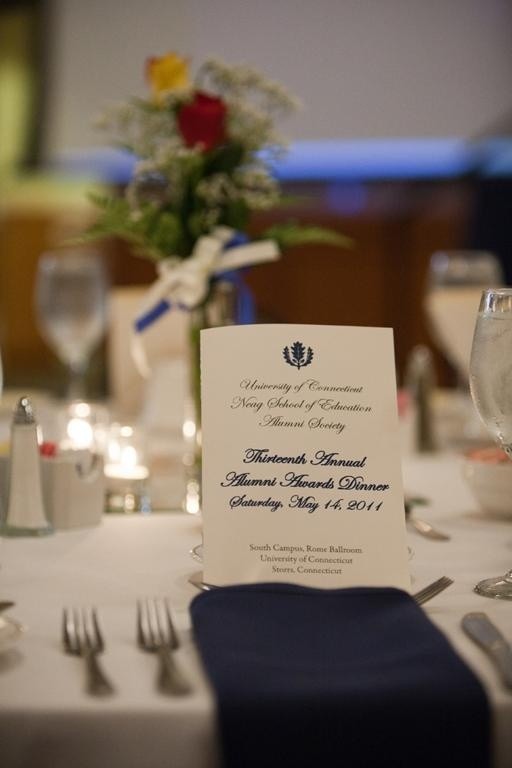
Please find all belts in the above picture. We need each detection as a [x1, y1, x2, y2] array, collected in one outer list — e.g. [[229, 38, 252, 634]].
[[421, 251, 497, 379], [466, 286, 512, 596], [37, 250, 102, 417]]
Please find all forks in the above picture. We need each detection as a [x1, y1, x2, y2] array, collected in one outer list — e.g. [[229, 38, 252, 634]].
[[137, 598, 189, 696], [59, 596, 108, 703], [413, 572, 452, 609]]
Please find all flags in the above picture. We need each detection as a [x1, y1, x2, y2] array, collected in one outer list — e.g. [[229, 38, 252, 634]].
[[181, 285, 242, 512]]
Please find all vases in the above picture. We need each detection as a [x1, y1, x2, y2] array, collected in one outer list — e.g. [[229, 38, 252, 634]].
[[55, 53, 353, 304]]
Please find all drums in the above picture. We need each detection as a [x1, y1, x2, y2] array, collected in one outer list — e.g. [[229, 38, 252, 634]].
[[456, 610, 512, 698]]
